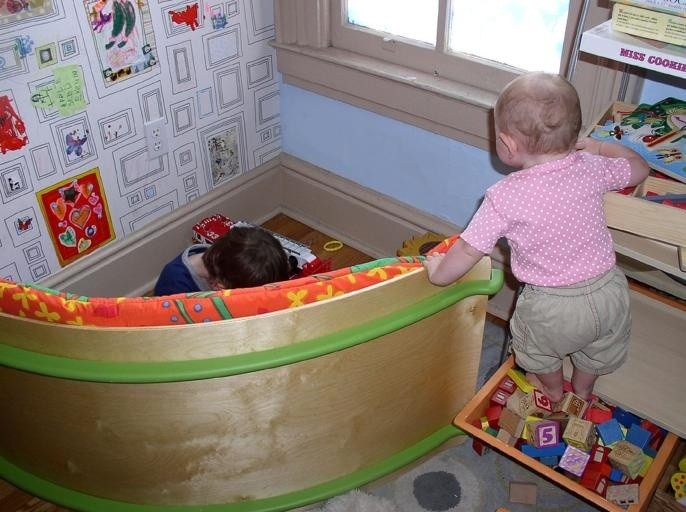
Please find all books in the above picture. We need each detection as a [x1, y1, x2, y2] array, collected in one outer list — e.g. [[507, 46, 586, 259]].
[[590, 95, 685, 184]]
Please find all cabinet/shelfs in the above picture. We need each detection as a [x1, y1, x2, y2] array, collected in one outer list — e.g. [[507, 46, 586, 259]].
[[449, 1, 686, 512]]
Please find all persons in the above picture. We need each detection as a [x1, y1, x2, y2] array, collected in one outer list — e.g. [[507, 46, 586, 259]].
[[423, 70, 651, 403], [153, 225, 291, 296]]
